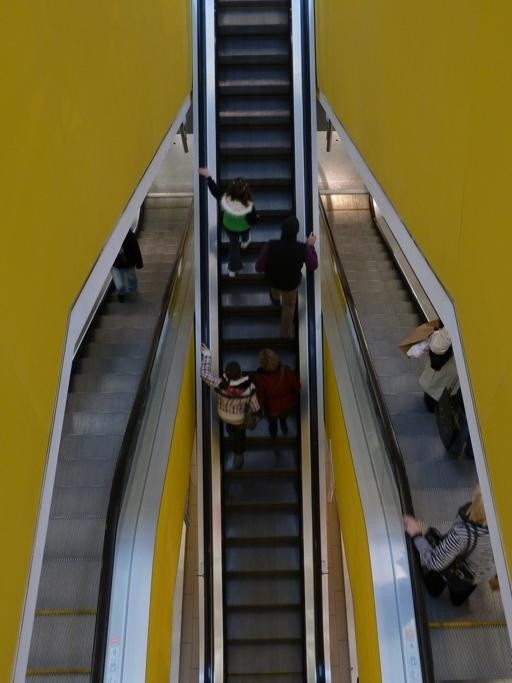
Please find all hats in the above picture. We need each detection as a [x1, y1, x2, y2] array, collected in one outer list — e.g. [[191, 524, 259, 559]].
[[428, 329, 452, 355], [259, 349, 280, 372]]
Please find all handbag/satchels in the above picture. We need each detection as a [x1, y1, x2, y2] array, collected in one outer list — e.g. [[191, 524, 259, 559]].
[[417, 522, 478, 594], [398, 319, 439, 359]]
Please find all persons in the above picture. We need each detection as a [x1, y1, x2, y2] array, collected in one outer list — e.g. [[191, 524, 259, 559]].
[[111, 228, 143, 303], [198, 166, 260, 278], [418, 324, 470, 413], [200, 342, 302, 470], [402, 480, 496, 606], [256, 216, 318, 339]]
